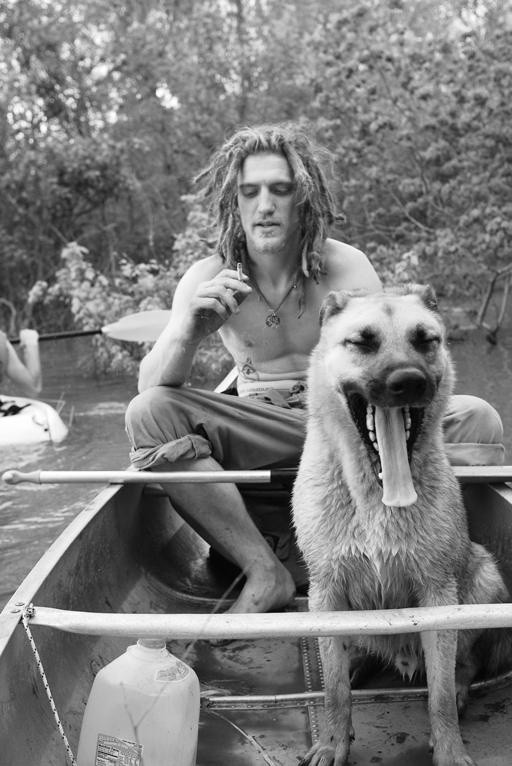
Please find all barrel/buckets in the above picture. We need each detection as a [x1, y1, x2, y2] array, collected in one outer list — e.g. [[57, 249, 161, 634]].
[[76, 636, 202, 766]]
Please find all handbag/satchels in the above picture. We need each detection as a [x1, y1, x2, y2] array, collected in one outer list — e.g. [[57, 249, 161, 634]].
[[207, 511, 311, 590]]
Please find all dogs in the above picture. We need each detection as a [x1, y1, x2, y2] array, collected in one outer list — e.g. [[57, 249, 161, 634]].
[[289, 282, 508, 766]]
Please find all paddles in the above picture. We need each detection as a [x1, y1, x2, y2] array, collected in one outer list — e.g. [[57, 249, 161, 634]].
[[7, 309, 168, 345]]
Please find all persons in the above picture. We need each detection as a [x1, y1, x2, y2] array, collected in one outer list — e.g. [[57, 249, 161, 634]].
[[0, 327, 44, 397], [122, 123, 505, 648]]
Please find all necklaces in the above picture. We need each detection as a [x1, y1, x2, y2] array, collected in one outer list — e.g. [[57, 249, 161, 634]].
[[246, 270, 299, 331]]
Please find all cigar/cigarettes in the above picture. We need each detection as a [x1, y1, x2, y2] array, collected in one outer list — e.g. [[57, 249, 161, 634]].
[[237, 262, 243, 280]]
